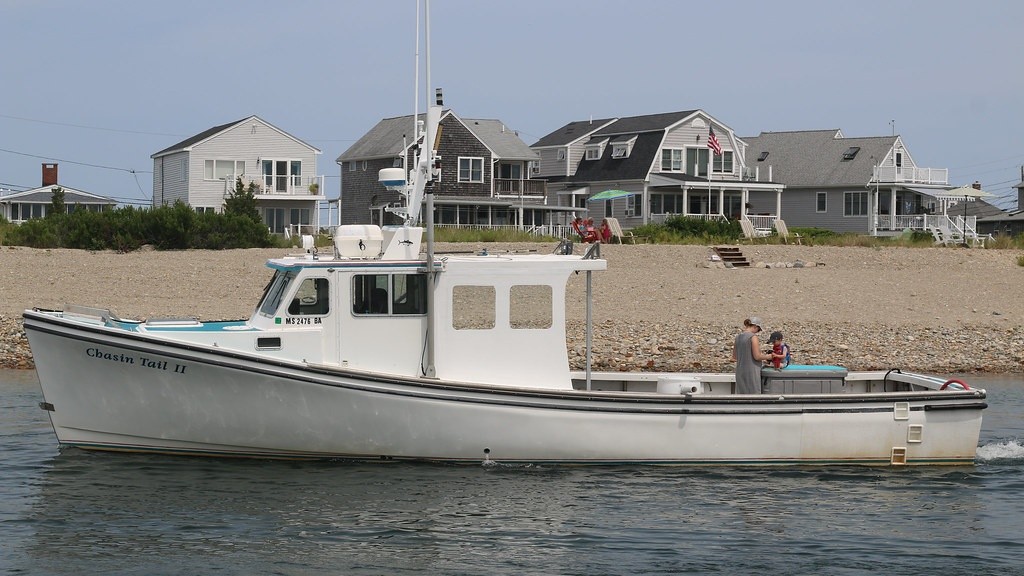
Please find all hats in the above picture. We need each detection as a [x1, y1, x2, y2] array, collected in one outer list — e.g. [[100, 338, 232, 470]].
[[748, 318, 763, 331], [767, 332, 783, 344]]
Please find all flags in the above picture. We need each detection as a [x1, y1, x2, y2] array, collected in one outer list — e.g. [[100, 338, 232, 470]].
[[707, 125, 722, 155]]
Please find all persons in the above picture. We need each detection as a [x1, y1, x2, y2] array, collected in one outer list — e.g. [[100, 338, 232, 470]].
[[765, 332, 791, 371], [575, 217, 613, 244], [733, 316, 772, 394]]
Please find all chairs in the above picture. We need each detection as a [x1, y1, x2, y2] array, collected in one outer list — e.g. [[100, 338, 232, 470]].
[[929, 224, 957, 248], [738, 220, 768, 245], [939, 225, 964, 248], [773, 220, 803, 245], [603, 217, 637, 245], [256, 178, 271, 194], [572, 221, 600, 243]]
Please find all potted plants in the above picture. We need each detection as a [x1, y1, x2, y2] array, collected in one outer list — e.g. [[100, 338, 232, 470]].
[[309, 183, 319, 195]]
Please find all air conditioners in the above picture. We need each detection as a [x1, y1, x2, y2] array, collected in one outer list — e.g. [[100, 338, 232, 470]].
[[624, 209, 634, 216]]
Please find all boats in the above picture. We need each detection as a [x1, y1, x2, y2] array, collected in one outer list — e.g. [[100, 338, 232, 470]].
[[21, 0, 987, 467]]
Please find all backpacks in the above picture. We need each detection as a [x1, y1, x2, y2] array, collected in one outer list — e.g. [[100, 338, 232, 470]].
[[781, 344, 790, 367]]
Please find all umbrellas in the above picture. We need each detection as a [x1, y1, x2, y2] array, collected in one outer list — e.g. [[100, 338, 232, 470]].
[[588, 189, 634, 218], [936, 185, 999, 243]]
